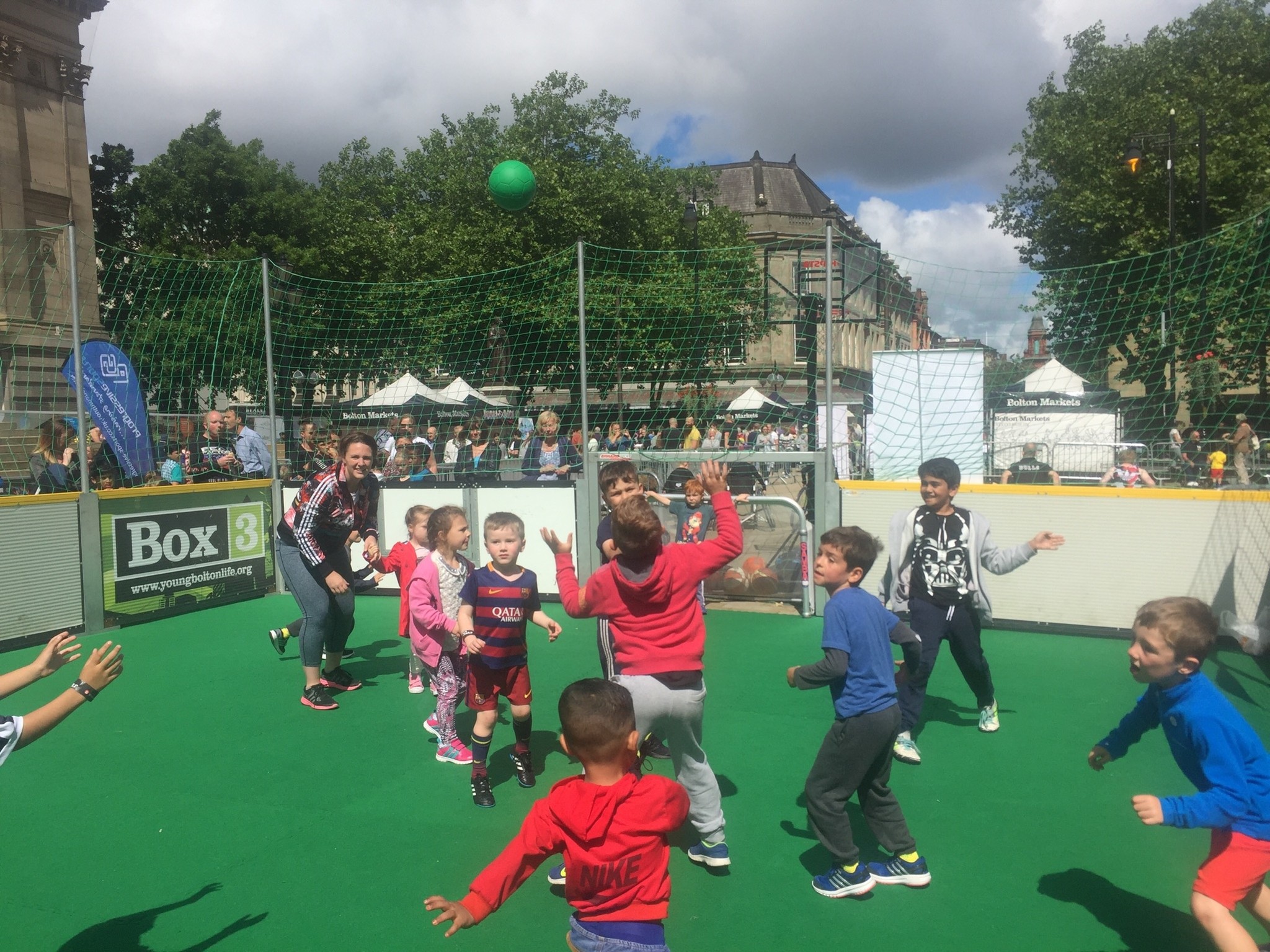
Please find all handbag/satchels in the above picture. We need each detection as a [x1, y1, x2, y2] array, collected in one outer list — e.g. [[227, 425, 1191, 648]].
[[1247, 426, 1261, 450]]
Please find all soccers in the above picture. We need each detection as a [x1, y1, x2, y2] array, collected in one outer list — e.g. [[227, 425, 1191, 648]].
[[489, 160, 536, 211]]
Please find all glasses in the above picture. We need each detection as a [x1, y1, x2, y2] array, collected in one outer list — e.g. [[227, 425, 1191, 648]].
[[401, 424, 413, 428]]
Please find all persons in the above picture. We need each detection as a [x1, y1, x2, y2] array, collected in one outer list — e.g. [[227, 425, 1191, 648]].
[[785, 524, 931, 897], [0, 407, 1270, 808], [427, 678, 691, 952], [539, 459, 734, 887], [0, 630, 126, 770], [1082, 596, 1270, 952], [274, 430, 381, 711]]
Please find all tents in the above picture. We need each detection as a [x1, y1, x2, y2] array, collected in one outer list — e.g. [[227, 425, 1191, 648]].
[[717, 385, 803, 423], [325, 371, 514, 420], [984, 360, 1126, 482]]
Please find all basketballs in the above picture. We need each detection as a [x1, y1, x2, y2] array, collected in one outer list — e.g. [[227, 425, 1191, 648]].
[[704, 556, 779, 594]]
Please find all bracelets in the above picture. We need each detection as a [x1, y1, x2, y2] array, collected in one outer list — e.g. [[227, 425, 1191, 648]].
[[461, 630, 476, 643], [71, 678, 98, 702]]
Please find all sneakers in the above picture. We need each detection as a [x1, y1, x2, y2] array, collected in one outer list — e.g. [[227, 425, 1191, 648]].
[[436, 739, 474, 765], [429, 680, 439, 696], [471, 773, 495, 808], [893, 735, 923, 764], [687, 836, 731, 867], [269, 628, 289, 656], [408, 672, 425, 694], [978, 697, 1000, 732], [322, 648, 354, 660], [423, 711, 441, 741], [300, 683, 338, 711], [509, 747, 536, 788], [867, 855, 931, 887], [547, 862, 565, 886], [320, 667, 362, 691], [811, 862, 876, 898]]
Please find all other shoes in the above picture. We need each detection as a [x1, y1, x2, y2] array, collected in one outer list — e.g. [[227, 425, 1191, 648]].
[[645, 732, 671, 758]]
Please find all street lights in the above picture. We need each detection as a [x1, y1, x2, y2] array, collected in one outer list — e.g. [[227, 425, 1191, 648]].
[[1123, 108, 1178, 429], [766, 359, 785, 392]]
